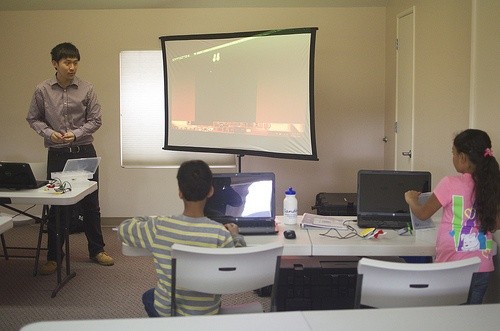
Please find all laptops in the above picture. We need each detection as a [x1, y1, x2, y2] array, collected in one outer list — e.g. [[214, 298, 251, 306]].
[[358, 170, 431, 229], [0, 162, 49, 189], [207, 173, 279, 234]]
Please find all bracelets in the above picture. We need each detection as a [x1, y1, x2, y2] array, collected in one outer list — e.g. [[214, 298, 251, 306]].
[[74, 133, 76, 143]]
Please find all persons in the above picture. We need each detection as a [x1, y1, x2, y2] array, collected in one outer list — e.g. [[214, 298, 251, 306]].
[[118, 160, 245, 317], [25, 42, 115, 275], [404, 129, 500, 305]]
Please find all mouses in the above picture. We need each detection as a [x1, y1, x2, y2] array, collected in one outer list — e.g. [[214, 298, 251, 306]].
[[284, 230, 296, 238]]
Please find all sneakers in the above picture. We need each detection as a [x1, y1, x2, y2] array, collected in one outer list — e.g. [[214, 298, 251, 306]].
[[40, 259, 62, 275], [91, 251, 114, 265]]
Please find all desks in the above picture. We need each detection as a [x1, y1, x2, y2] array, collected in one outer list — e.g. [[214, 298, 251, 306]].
[[121, 213, 500, 311], [19, 303, 500, 331], [0, 179, 98, 298]]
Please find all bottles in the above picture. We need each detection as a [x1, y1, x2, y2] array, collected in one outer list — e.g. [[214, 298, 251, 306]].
[[283, 188, 298, 225]]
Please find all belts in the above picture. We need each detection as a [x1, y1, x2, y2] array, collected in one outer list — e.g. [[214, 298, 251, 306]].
[[50, 145, 91, 153]]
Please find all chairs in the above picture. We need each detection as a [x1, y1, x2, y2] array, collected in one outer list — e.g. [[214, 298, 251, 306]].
[[0, 162, 51, 278], [170, 240, 285, 315], [350, 254, 483, 308]]
